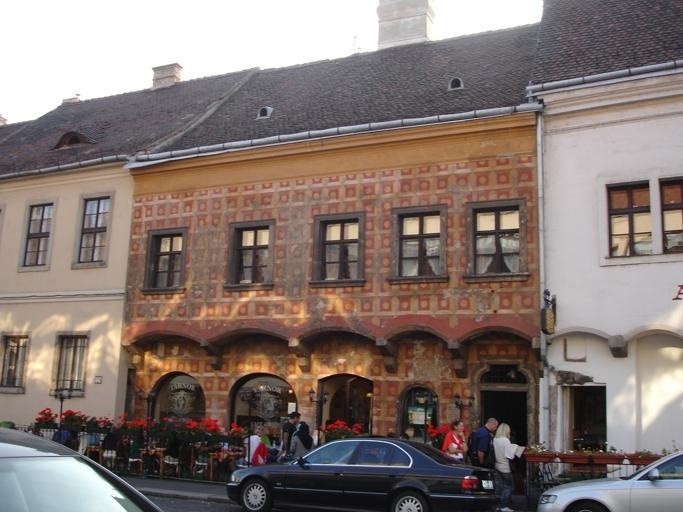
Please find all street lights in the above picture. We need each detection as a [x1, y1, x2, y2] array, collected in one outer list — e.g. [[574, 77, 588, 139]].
[[309, 389, 329, 446], [136, 389, 158, 479], [240, 389, 261, 468], [416, 392, 476, 444], [54, 387, 72, 444]]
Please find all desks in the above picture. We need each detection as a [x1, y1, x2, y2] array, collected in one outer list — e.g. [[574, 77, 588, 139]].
[[138, 448, 167, 479], [209, 451, 238, 484], [86, 446, 105, 466], [567, 466, 621, 481]]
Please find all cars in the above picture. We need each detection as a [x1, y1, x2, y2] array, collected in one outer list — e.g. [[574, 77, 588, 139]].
[[224, 437, 498, 512], [536, 449, 683, 511], [0, 426, 164, 512]]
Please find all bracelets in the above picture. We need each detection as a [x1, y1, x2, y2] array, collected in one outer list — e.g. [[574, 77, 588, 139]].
[[461, 439, 465, 441]]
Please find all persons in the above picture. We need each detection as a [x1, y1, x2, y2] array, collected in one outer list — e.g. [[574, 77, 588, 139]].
[[282, 411, 301, 451], [312, 421, 329, 447], [493, 422, 529, 512], [467, 417, 499, 469], [287, 421, 314, 460], [23, 415, 286, 485], [439, 418, 468, 462]]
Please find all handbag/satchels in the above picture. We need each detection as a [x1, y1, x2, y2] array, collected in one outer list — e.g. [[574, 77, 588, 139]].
[[251, 442, 267, 466]]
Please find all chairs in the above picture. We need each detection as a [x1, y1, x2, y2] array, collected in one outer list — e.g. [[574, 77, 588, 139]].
[[164, 460, 179, 479], [129, 457, 142, 477], [193, 459, 208, 482], [530, 461, 571, 497], [103, 449, 116, 469]]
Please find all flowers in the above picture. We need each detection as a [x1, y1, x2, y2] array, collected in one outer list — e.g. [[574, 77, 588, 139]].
[[33, 406, 249, 446], [322, 419, 364, 442], [424, 420, 452, 451]]
